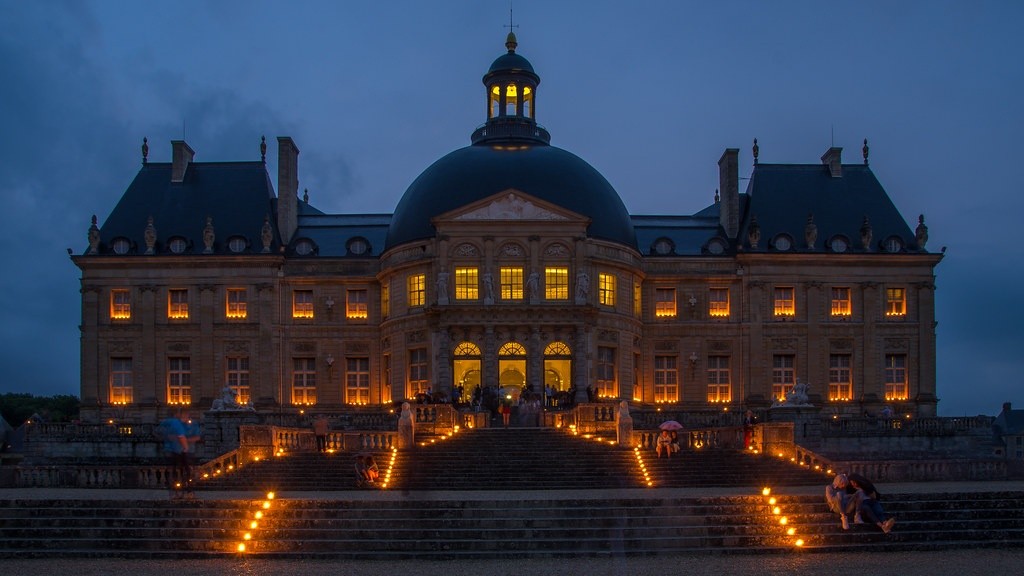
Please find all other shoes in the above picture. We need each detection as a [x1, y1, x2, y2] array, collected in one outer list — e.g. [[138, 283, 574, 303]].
[[364, 479, 374, 482], [503, 425, 510, 429], [882, 518, 895, 533]]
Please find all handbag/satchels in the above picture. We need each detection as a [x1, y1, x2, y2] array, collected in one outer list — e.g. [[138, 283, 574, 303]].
[[498, 403, 504, 414]]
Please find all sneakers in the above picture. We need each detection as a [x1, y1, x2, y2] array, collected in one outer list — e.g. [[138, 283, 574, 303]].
[[854, 512, 864, 523], [841, 516, 849, 529]]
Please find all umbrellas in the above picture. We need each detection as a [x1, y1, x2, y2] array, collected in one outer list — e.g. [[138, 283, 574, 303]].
[[659, 421, 683, 431]]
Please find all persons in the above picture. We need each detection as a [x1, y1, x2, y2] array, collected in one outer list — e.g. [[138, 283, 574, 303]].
[[154, 407, 189, 500], [165, 407, 200, 499], [881, 406, 891, 429], [221, 382, 234, 407], [354, 456, 366, 486], [848, 474, 895, 533], [312, 414, 330, 452], [742, 410, 757, 451], [416, 382, 600, 427], [670, 431, 680, 452], [825, 474, 865, 529], [362, 456, 379, 482], [435, 266, 592, 304], [656, 430, 671, 458], [788, 378, 810, 398]]
